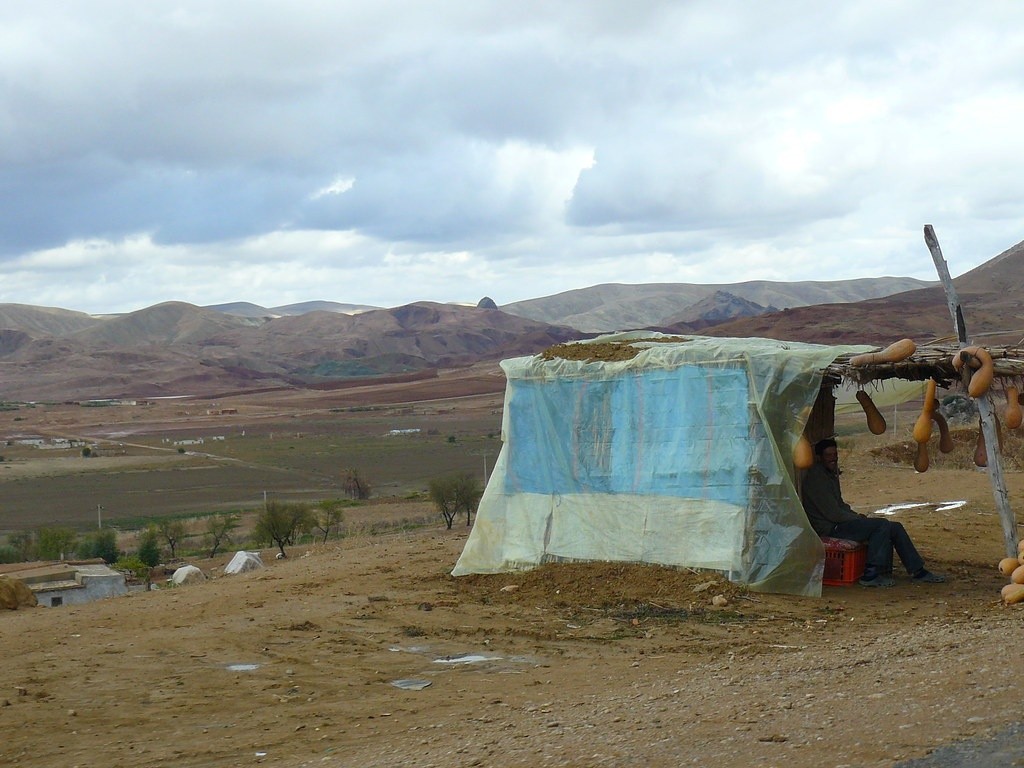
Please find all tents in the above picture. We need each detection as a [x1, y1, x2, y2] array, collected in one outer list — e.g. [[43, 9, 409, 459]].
[[224, 551, 264, 574], [172, 564, 206, 585]]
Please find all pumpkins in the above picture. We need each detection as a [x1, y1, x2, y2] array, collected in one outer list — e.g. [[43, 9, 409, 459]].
[[998, 539, 1024, 603], [791, 406, 813, 468], [847, 338, 1022, 472]]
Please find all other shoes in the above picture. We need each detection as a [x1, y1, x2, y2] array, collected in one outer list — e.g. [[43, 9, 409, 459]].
[[912, 572, 945, 583], [859, 576, 895, 587]]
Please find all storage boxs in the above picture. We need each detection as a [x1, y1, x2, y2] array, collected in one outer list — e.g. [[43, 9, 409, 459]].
[[822, 544, 864, 588]]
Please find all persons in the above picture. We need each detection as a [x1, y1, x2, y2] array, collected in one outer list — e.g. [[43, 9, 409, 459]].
[[802, 439, 945, 587]]
